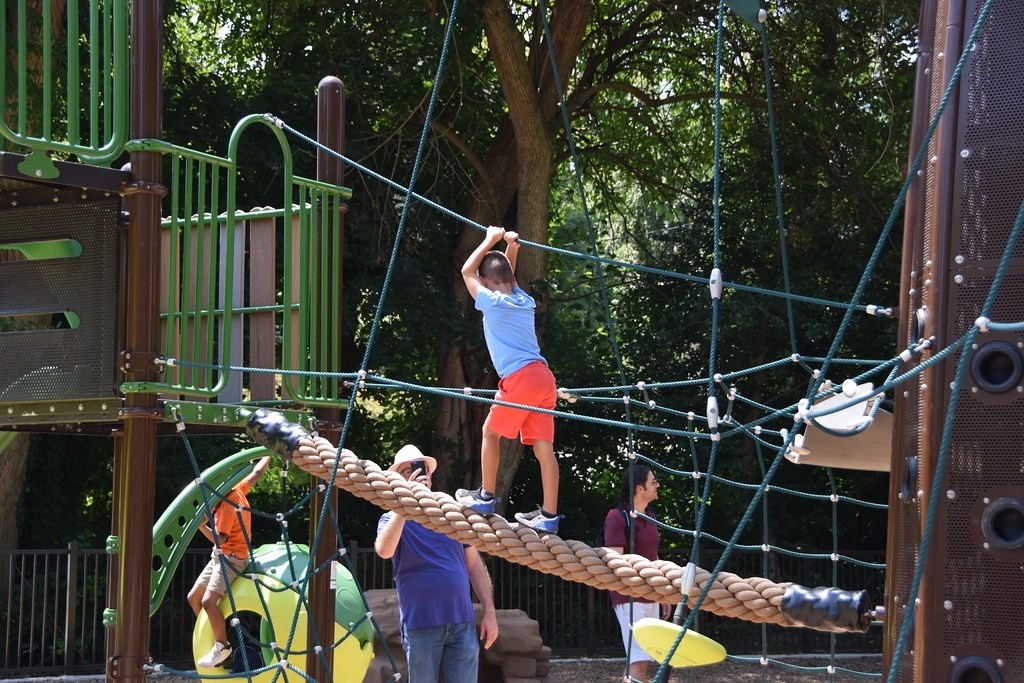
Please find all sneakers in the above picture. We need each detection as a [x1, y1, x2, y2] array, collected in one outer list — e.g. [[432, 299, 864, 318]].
[[198, 641, 233, 668], [455, 487, 500, 516], [514, 504, 565, 535]]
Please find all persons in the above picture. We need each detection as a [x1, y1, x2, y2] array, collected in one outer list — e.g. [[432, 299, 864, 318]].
[[455, 226, 559, 533], [374, 444, 499, 683], [605, 461, 672, 683], [187, 453, 271, 668]]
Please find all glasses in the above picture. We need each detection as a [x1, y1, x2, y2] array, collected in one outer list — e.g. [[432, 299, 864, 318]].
[[647, 476, 659, 486], [399, 466, 429, 477]]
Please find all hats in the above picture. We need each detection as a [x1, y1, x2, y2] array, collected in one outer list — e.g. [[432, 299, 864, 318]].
[[388, 444, 437, 475], [620, 464, 649, 495]]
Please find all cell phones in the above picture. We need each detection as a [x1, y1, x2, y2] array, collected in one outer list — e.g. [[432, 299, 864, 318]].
[[411, 460, 427, 487]]
